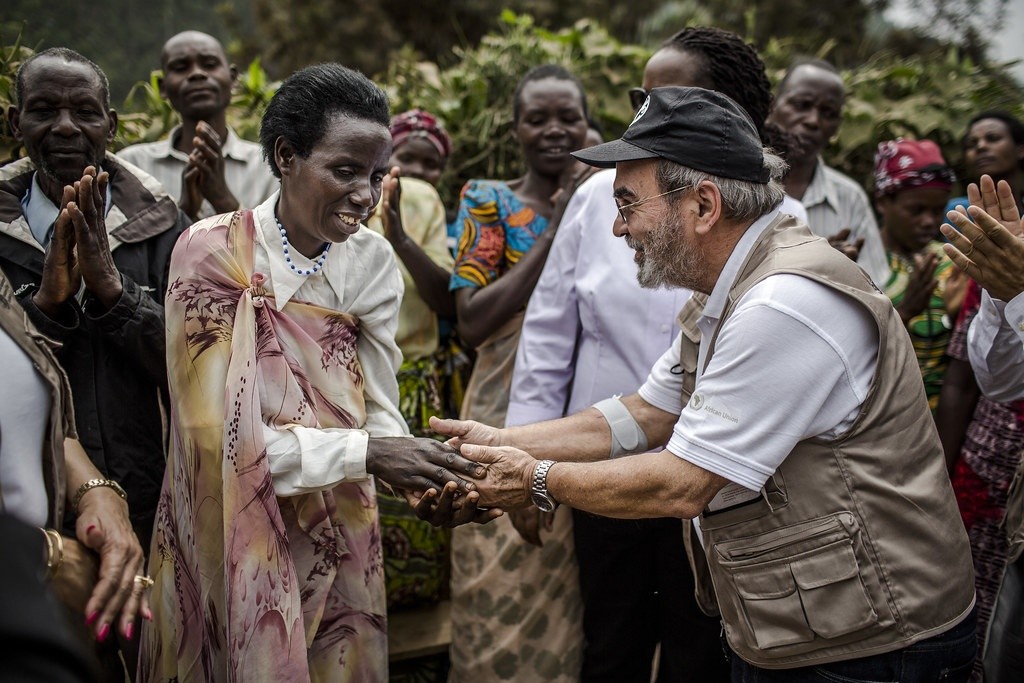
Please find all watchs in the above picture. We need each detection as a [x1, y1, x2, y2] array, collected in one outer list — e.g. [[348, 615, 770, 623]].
[[71, 479, 127, 514], [529, 460, 563, 513]]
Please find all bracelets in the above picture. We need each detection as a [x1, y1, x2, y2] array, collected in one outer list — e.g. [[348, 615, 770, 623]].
[[39, 526, 64, 576]]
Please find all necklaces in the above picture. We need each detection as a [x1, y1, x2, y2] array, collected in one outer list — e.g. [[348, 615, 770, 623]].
[[276, 220, 329, 275]]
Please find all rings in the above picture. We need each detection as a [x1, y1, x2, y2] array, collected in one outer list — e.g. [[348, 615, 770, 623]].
[[134, 574, 155, 590]]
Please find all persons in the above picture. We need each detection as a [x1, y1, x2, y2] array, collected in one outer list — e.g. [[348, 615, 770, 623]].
[[431, 87, 979, 683], [506, 28, 773, 682], [386, 107, 452, 186], [448, 65, 596, 682], [132, 63, 487, 683], [765, 60, 892, 292], [865, 137, 972, 457], [940, 173, 1024, 683], [2, 46, 194, 573], [964, 113, 1024, 217], [113, 33, 282, 221], [0, 268, 154, 683]]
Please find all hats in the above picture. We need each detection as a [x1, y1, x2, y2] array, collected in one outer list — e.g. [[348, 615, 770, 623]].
[[389, 110, 451, 161], [866, 139, 955, 200], [570, 85, 770, 186]]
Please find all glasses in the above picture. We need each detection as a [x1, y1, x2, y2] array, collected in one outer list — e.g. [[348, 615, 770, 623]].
[[628, 87, 650, 115], [614, 182, 697, 226]]
